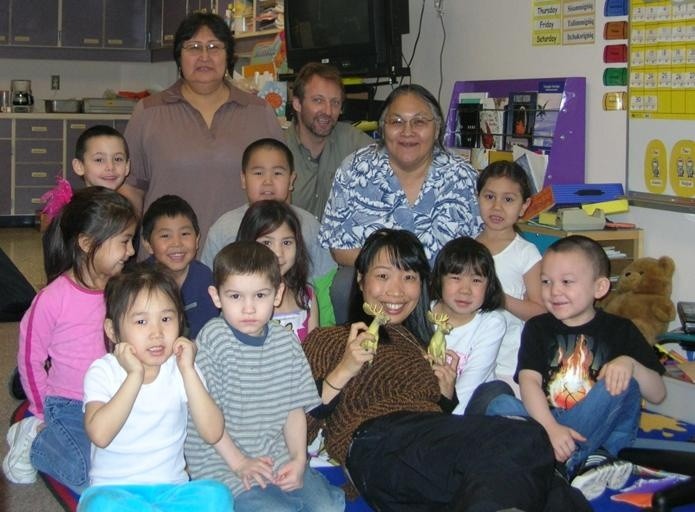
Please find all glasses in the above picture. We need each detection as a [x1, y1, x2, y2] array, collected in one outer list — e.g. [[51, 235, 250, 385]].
[[182, 40, 226, 55], [383, 115, 434, 128]]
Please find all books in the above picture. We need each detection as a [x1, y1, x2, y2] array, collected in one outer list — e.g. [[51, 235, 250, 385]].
[[453, 93, 563, 191]]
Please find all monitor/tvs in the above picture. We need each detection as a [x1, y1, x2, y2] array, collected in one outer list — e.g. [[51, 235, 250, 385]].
[[283, 0, 410, 75]]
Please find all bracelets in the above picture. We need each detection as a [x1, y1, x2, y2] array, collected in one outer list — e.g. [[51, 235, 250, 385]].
[[323, 375, 344, 391]]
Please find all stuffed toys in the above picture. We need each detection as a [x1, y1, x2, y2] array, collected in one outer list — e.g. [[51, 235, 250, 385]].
[[599, 255, 676, 347]]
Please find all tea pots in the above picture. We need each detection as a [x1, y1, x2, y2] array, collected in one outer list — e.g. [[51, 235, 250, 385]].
[[13, 94, 28, 105]]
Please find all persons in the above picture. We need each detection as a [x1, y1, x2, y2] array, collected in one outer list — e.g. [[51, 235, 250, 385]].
[[474, 161, 546, 399], [183, 241, 346, 512], [301, 230, 554, 512], [200, 137, 337, 285], [486, 235, 667, 502], [1, 179, 138, 486], [316, 83, 486, 270], [71, 124, 131, 190], [116, 12, 290, 262], [81, 268, 233, 512], [129, 194, 219, 340], [429, 236, 507, 416], [282, 62, 375, 224]]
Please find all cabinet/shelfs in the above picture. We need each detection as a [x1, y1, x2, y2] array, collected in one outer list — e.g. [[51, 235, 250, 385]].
[[0, 113, 133, 215], [514, 216, 644, 312], [442, 78, 585, 194], [152, 0, 232, 61], [0, 0, 152, 63], [233, 1, 285, 59]]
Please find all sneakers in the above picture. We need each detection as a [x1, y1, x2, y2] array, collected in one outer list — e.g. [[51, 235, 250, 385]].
[[2, 415, 44, 485], [570, 449, 634, 502]]
